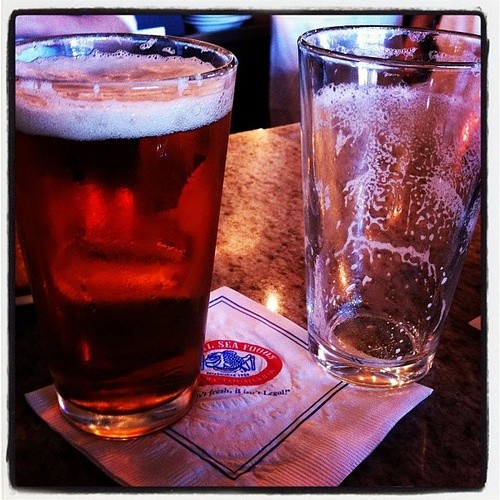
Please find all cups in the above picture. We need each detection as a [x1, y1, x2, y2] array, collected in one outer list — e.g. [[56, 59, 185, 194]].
[[297, 25, 482, 392], [14, 33, 239, 439]]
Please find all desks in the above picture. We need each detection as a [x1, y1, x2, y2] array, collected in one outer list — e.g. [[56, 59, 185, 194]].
[[14, 121, 480, 494]]
